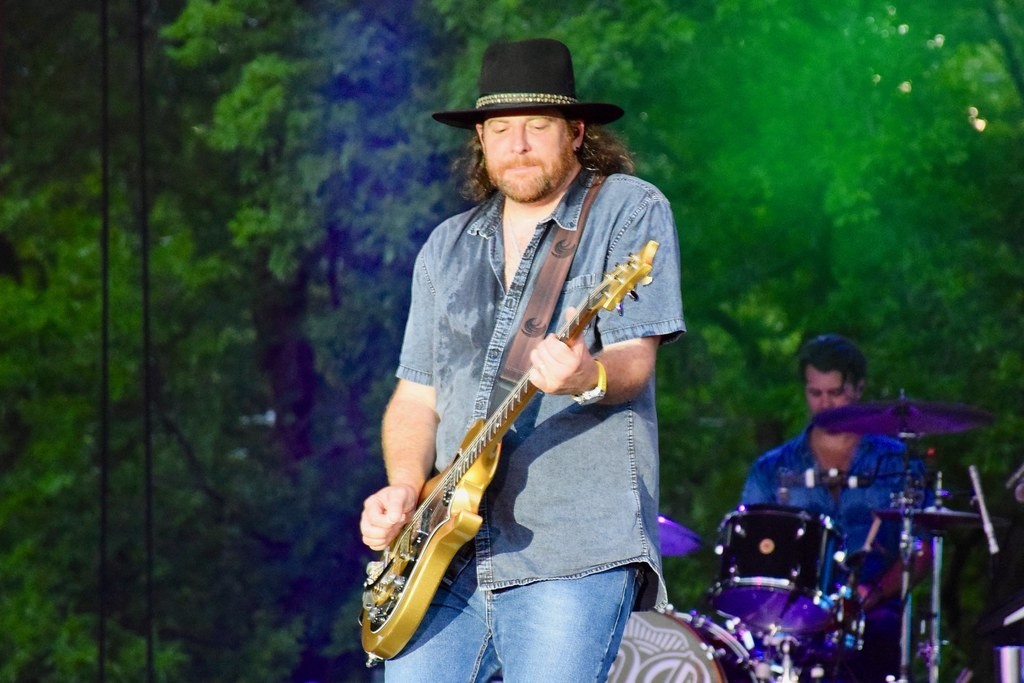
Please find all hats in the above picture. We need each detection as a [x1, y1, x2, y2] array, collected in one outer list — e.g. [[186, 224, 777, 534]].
[[431, 38, 625, 130]]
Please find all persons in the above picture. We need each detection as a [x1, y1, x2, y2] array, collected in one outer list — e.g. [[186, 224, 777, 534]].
[[359, 39, 687, 683], [738, 334, 934, 683]]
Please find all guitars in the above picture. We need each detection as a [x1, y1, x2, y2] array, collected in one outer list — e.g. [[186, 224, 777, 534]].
[[357, 235, 661, 667]]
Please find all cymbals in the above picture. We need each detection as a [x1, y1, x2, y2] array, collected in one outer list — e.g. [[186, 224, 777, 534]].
[[807, 397, 996, 438], [868, 498, 1008, 533], [657, 514, 701, 558]]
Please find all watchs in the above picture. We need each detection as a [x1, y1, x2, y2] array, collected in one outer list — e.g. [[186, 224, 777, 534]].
[[571, 360, 607, 407]]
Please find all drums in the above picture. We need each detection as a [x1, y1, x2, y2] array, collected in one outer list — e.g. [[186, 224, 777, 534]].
[[604, 607, 757, 683], [712, 503, 854, 637]]
